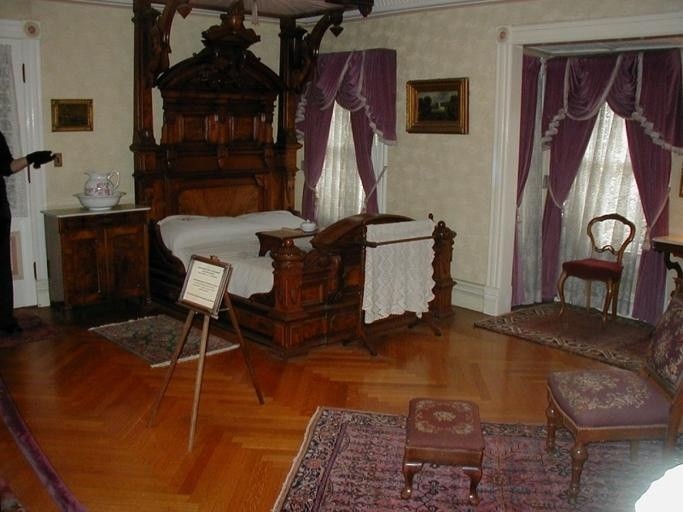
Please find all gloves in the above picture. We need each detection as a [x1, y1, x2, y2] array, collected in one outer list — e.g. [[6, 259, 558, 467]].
[[26, 150, 57, 169]]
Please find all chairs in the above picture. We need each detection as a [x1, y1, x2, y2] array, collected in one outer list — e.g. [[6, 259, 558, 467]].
[[558, 213, 635, 322], [547, 288, 683, 506]]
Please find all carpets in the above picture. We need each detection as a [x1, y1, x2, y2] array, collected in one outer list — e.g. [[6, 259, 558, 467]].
[[88, 314, 240, 368], [268, 406, 683, 512], [473, 297, 656, 374]]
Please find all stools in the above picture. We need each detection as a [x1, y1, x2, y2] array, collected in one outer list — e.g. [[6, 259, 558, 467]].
[[398, 399, 486, 505]]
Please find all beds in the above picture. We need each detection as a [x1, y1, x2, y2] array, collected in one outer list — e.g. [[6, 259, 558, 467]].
[[130, 0, 462, 358]]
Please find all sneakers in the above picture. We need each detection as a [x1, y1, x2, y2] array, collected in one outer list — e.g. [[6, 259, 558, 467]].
[[0, 322, 23, 338]]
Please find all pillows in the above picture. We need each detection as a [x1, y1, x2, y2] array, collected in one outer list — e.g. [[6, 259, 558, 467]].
[[236, 209, 308, 233], [160, 215, 245, 250]]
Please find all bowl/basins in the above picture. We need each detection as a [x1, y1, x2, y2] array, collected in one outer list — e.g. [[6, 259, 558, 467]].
[[72, 191, 127, 211]]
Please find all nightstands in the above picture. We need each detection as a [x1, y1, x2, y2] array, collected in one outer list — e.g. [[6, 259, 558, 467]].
[[41, 202, 153, 325]]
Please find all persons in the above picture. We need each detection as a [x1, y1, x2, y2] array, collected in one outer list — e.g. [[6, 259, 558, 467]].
[[0, 128, 57, 337]]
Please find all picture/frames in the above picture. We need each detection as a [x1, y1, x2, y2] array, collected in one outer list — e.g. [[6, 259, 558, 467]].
[[50, 99, 93, 132], [406, 77, 469, 134]]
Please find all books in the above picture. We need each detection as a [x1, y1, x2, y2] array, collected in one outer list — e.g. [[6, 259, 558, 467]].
[[652, 235, 683, 246]]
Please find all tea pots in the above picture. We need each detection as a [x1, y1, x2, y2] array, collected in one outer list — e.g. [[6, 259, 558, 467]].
[[84, 170, 120, 195]]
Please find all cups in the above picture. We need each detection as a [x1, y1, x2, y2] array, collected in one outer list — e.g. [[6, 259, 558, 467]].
[[300, 221, 317, 232]]
